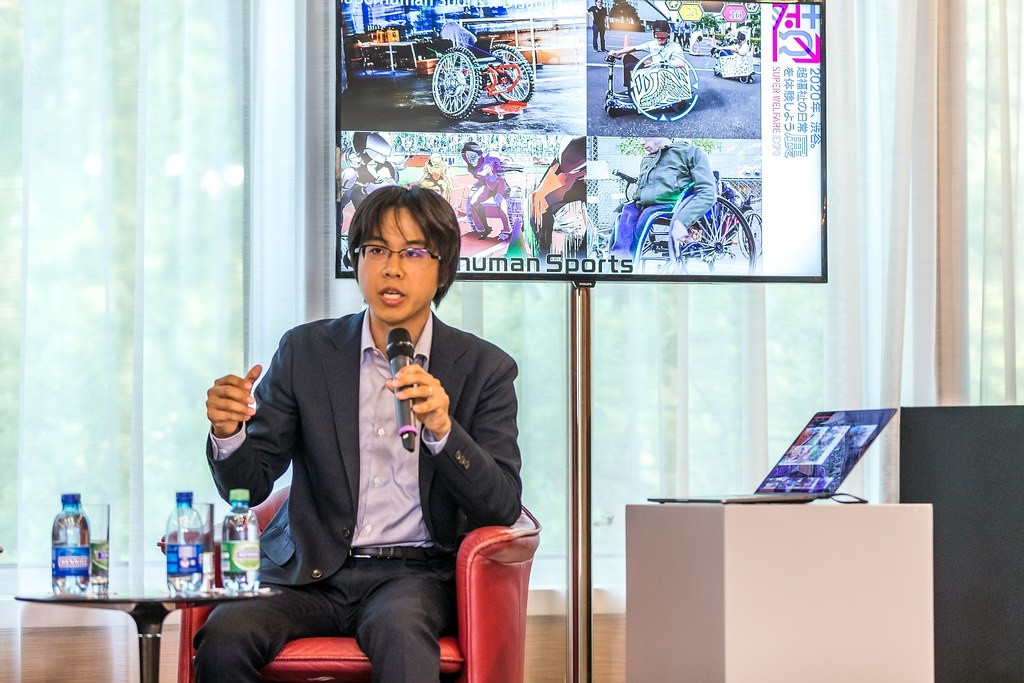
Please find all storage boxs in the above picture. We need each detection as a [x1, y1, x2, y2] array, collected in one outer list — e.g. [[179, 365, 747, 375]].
[[418, 58, 440, 75]]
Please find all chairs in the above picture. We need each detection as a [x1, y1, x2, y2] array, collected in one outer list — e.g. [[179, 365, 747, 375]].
[[173, 484, 539, 683]]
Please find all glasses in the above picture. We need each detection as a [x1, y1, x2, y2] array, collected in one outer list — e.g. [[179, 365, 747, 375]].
[[353, 245, 440, 267]]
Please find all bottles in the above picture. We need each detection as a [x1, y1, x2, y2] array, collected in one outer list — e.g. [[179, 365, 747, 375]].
[[164, 492, 202, 593], [221, 488, 262, 593], [49, 494, 90, 597]]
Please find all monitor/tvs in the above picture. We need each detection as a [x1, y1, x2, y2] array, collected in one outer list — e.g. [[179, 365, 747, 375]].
[[336, 0, 828, 284]]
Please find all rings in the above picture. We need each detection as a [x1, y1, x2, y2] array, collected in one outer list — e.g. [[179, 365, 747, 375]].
[[428, 387, 432, 398]]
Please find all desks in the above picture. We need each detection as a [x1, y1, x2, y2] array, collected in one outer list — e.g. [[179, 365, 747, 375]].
[[14, 581, 286, 682], [360, 41, 419, 73]]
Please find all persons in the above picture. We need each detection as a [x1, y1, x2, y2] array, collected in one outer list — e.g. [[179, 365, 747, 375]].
[[191, 185, 522, 683], [586, 0, 609, 53], [673, 19, 692, 50], [711, 27, 749, 58], [607, 136, 717, 259], [609, 20, 685, 103]]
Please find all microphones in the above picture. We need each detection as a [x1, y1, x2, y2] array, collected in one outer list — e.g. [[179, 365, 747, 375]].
[[386, 328, 418, 452]]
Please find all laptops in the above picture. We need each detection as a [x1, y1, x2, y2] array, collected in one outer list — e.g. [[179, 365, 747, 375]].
[[647, 408, 897, 504]]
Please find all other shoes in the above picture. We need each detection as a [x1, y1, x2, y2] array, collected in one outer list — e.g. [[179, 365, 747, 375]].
[[593, 48, 599, 52], [602, 48, 608, 53]]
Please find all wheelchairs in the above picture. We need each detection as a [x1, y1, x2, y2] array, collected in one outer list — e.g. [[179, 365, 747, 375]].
[[602, 171, 762, 277]]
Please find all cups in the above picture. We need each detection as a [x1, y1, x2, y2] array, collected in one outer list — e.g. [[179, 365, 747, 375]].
[[79, 504, 110, 595], [177, 502, 214, 592]]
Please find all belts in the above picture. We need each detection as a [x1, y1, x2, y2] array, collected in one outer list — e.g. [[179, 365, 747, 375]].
[[349, 546, 435, 561]]
